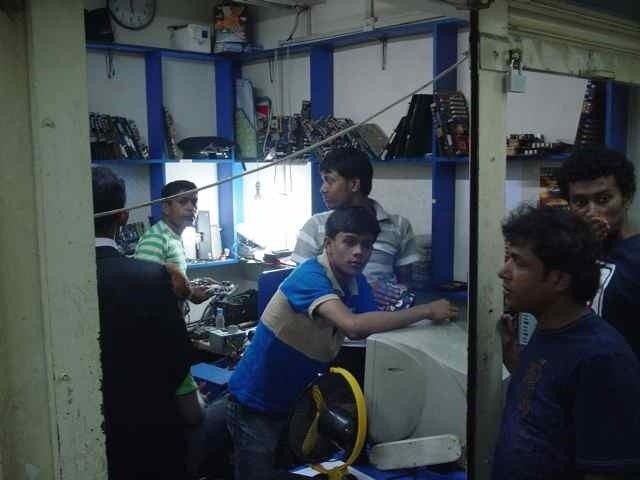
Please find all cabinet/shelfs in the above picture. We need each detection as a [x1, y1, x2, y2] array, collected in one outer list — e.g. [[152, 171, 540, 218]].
[[86, 16, 468, 302]]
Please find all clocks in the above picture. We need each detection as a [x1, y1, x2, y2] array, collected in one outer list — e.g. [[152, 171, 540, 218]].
[[110, 0, 156, 30]]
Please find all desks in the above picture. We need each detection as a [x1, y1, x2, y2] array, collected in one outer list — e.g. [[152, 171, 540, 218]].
[[287, 448, 469, 480], [187, 319, 244, 359]]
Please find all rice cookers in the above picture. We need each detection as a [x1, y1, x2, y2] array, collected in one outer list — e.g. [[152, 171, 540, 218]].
[[168, 23, 212, 55]]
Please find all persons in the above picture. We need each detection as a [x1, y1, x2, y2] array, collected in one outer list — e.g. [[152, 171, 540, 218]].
[[493, 196, 638, 479], [134, 180, 213, 426], [228, 201, 459, 480], [291, 149, 422, 372], [93, 166, 197, 480], [498, 143, 640, 369]]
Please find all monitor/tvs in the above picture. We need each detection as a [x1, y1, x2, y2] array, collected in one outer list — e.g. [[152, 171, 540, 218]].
[[361, 319, 513, 471]]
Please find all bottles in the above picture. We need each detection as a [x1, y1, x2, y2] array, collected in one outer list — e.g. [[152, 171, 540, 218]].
[[215, 307, 225, 329]]
